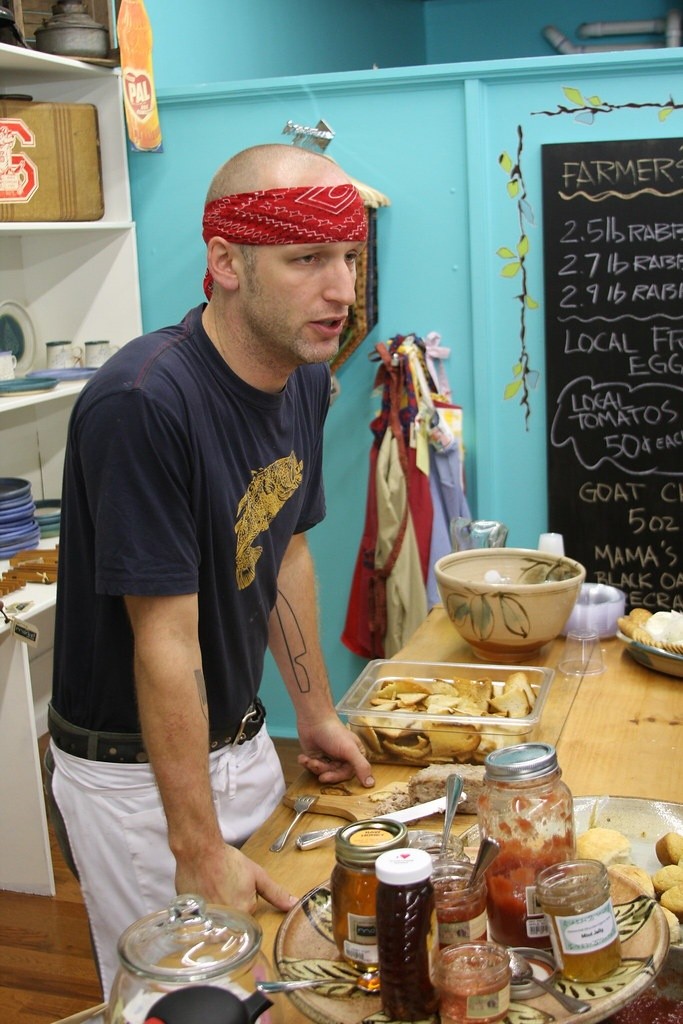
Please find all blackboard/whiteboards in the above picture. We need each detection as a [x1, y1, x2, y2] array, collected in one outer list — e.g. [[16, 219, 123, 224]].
[[541, 137, 683, 615]]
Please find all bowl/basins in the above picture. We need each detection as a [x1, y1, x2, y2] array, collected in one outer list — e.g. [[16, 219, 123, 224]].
[[615, 630, 683, 678], [335, 657, 555, 768], [435, 548, 586, 663]]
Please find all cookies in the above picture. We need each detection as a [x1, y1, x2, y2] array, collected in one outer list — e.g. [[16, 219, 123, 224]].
[[617, 608, 683, 655]]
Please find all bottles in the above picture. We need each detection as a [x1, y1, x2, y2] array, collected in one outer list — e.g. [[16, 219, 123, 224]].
[[104, 892, 280, 1024], [331, 743, 621, 1024]]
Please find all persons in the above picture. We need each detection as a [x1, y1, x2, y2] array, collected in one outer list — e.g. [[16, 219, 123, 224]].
[[48, 143, 375, 1003]]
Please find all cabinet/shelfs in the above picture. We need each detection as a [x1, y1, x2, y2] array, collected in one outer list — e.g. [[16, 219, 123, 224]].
[[0, 44, 141, 897]]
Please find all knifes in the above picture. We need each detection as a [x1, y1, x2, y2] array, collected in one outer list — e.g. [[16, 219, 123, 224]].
[[295, 792, 467, 851]]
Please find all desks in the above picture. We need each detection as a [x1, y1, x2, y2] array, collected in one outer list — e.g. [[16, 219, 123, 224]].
[[162, 605, 682, 1023]]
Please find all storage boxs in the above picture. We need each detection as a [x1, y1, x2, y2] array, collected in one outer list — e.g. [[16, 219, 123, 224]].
[[10, 0, 120, 67]]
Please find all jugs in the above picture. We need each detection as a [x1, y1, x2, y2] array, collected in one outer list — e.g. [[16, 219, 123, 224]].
[[145, 985, 271, 1023]]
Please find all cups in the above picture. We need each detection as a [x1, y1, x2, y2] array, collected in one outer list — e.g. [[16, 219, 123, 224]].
[[84, 340, 120, 368], [558, 631, 607, 678], [537, 533, 565, 559], [46, 341, 82, 370], [0, 350, 17, 381]]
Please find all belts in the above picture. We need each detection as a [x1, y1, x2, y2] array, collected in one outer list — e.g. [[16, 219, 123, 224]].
[[47, 697, 266, 765]]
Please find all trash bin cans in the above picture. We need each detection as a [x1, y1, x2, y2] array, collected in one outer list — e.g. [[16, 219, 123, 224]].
[[44, 746, 81, 883]]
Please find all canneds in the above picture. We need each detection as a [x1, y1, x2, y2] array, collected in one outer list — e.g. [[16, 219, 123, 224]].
[[331, 741, 621, 1024]]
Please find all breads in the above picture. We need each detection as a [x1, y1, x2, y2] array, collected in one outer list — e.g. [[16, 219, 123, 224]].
[[347, 673, 539, 765], [570, 824, 683, 945], [369, 789, 421, 827], [407, 765, 487, 815]]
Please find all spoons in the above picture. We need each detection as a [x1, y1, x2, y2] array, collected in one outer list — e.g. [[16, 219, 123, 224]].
[[256, 970, 379, 993], [506, 949, 592, 1015]]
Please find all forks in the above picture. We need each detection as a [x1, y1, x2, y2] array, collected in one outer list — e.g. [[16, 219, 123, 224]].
[[270, 795, 319, 852]]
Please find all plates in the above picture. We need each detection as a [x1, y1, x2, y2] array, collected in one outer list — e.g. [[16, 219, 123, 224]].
[[458, 795, 682, 952], [27, 367, 98, 379], [1, 380, 61, 391], [0, 302, 36, 377], [1, 477, 62, 560], [273, 846, 669, 1024]]
